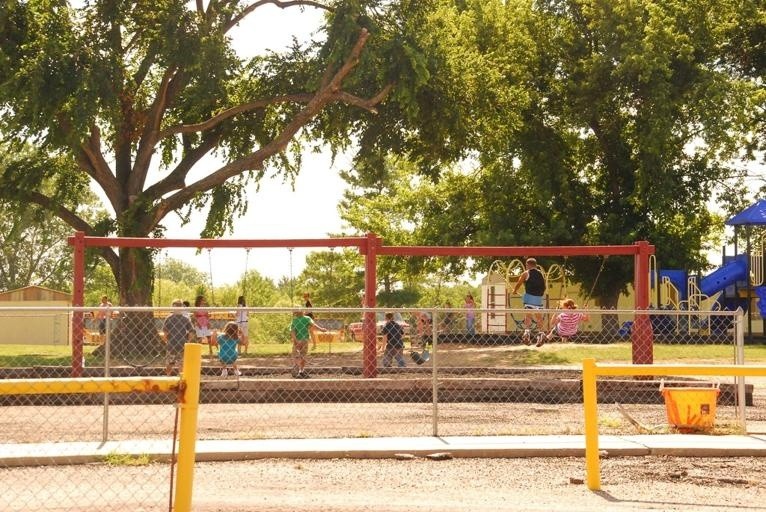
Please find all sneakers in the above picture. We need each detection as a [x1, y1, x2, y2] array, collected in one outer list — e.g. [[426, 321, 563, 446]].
[[220, 368, 306, 381], [521, 331, 568, 347]]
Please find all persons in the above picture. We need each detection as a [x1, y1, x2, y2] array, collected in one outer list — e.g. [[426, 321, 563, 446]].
[[211, 321, 247, 377], [465, 294, 476, 333], [511, 256, 547, 346], [291, 304, 327, 379], [382, 314, 405, 367], [444, 301, 453, 332], [302, 292, 317, 349], [550, 298, 590, 344], [163, 302, 194, 377], [99, 296, 115, 346], [416, 312, 433, 335], [192, 296, 213, 354], [237, 295, 249, 355]]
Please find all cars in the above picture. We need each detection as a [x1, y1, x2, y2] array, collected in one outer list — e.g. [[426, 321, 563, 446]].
[[349, 308, 412, 343]]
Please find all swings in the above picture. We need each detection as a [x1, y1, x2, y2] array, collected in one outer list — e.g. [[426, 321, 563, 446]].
[[552, 256, 607, 337], [289, 249, 336, 365], [207, 248, 250, 365], [117, 246, 162, 367], [408, 254, 447, 365]]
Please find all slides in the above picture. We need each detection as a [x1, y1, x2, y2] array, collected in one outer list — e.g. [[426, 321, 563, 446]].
[[620, 262, 745, 335]]
